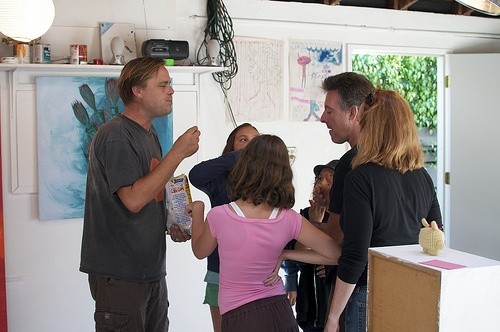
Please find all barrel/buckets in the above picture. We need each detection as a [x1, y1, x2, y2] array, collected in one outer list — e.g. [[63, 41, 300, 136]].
[[13, 43, 31, 64], [32, 44, 51, 63], [69, 44, 88, 64]]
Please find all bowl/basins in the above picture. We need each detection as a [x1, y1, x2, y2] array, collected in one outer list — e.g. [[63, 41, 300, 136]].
[[0, 57, 20, 64]]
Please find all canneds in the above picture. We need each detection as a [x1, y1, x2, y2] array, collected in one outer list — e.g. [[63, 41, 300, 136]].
[[69, 45, 87, 65], [15, 43, 52, 63]]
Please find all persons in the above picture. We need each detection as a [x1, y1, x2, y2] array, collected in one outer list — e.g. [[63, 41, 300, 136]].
[[186, 134, 342, 332], [79, 56, 201, 332], [188, 123, 298, 332], [299, 159, 341, 332], [323, 88, 443, 332], [306, 72, 376, 332]]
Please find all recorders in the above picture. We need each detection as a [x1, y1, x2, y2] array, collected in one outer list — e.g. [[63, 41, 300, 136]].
[[141, 39, 189, 60]]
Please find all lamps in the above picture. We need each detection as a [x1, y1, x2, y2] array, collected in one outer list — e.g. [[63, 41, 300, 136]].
[[456, 0, 500, 16]]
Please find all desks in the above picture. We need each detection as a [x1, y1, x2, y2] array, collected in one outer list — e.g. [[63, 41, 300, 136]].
[[365, 245, 500, 332]]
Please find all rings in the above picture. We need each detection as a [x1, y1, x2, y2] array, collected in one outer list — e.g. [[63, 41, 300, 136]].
[[176, 239, 179, 241]]
